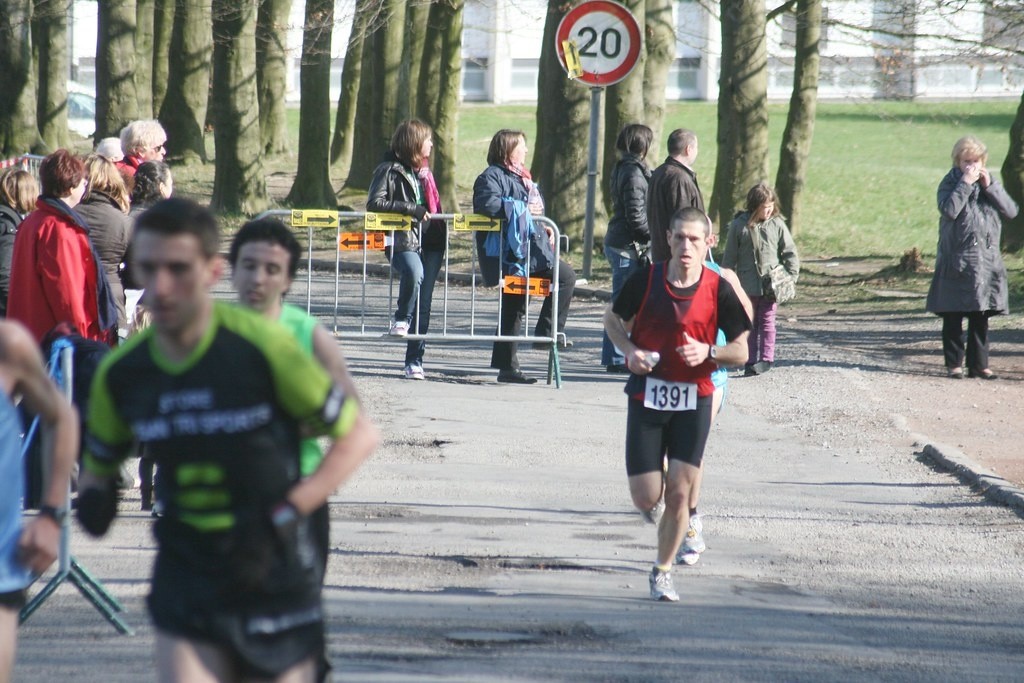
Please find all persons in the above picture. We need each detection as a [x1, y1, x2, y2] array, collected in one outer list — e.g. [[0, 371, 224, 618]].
[[77, 198, 376, 683], [676, 217, 753, 564], [473, 129, 575, 383], [604, 207, 753, 601], [230, 218, 357, 582], [925, 136, 1018, 380], [601, 124, 653, 371], [0, 320, 76, 683], [366, 119, 445, 379], [647, 129, 717, 263], [722, 185, 800, 374], [0, 121, 173, 511]]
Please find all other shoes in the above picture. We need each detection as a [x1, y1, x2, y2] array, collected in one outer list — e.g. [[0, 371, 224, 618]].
[[607, 364, 631, 373], [947, 366, 964, 378], [745, 364, 756, 376], [750, 360, 770, 375], [967, 367, 997, 380]]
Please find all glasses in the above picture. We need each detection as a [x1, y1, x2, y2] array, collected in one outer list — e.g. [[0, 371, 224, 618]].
[[80, 179, 88, 186], [149, 145, 162, 153]]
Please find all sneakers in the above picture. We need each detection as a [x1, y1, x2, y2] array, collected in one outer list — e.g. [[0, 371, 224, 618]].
[[681, 514, 706, 554], [640, 463, 667, 525], [406, 363, 424, 380], [391, 322, 410, 336], [675, 545, 699, 565], [649, 566, 679, 602], [533, 340, 573, 350], [497, 371, 537, 384]]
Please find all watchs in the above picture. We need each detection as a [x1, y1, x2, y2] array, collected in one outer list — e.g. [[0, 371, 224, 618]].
[[41, 506, 66, 525], [706, 343, 716, 360]]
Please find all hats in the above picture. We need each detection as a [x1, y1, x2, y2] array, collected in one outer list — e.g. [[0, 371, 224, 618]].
[[95, 137, 125, 162]]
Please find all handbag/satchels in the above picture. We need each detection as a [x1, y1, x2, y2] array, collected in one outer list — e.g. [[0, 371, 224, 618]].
[[762, 265, 795, 303]]
